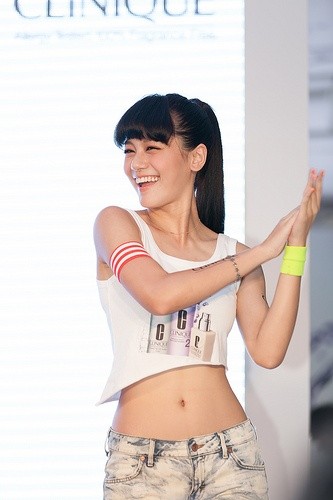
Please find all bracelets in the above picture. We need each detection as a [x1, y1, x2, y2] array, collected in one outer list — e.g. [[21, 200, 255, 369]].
[[281, 245, 306, 276], [227, 255, 243, 282]]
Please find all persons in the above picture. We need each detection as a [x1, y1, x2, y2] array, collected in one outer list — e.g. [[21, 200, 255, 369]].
[[95, 94, 325, 498]]
